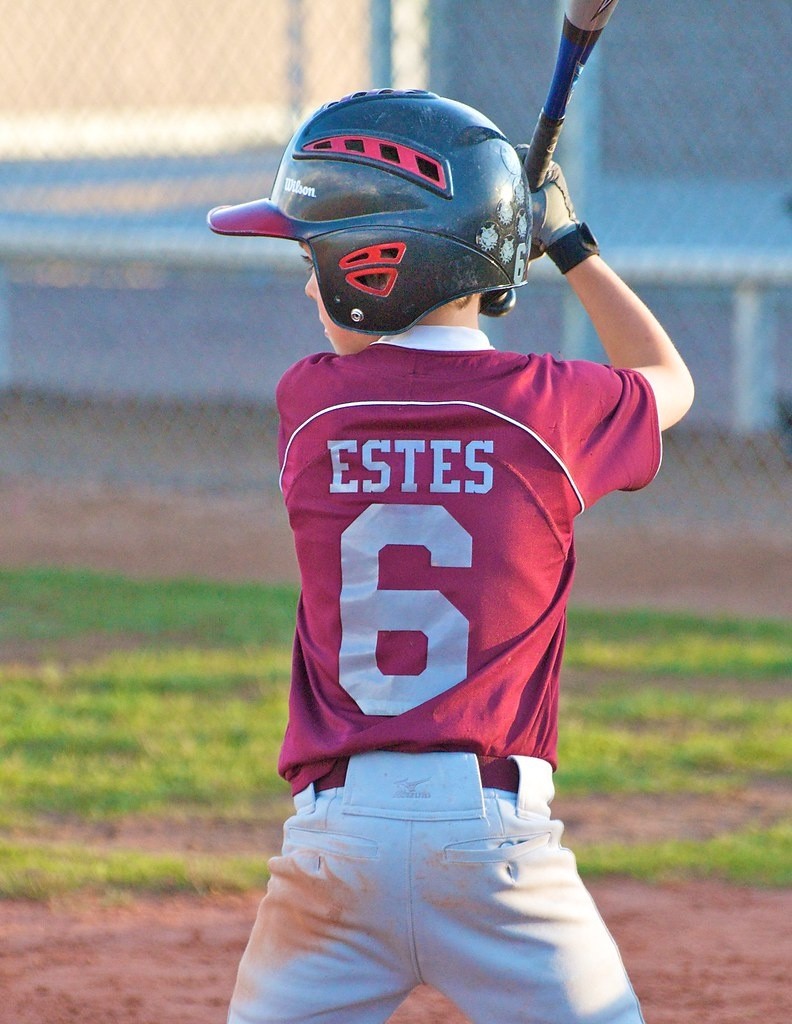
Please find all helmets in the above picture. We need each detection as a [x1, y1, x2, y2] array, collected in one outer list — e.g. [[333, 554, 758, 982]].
[[208, 90, 534, 335]]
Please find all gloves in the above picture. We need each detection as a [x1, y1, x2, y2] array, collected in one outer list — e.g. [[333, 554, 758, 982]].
[[511, 138, 601, 274]]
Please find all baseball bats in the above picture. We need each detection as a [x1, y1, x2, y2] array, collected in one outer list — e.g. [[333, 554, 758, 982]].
[[478, 1, 619, 319]]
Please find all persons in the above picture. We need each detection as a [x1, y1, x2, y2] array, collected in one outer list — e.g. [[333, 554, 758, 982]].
[[205, 89, 694, 1024]]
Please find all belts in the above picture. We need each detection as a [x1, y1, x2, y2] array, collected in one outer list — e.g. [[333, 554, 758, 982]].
[[314, 748, 518, 791]]
